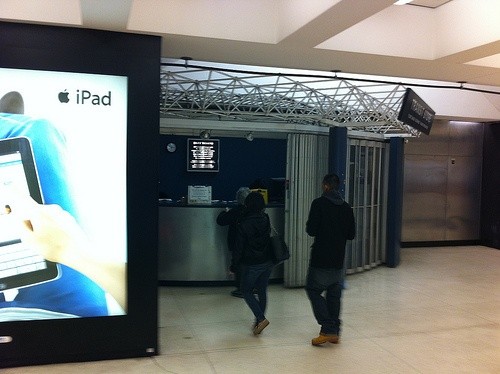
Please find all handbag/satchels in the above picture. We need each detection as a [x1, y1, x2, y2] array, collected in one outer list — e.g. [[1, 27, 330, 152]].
[[266, 233, 289, 266]]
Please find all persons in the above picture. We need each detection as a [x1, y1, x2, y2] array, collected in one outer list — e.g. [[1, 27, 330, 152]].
[[216, 187, 258, 297], [306, 174, 356, 346], [227, 191, 271, 336]]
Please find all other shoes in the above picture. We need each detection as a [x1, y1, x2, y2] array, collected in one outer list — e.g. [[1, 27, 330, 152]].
[[231, 289, 245, 298], [253, 318, 270, 335], [330, 335, 339, 344], [311, 333, 338, 345]]
